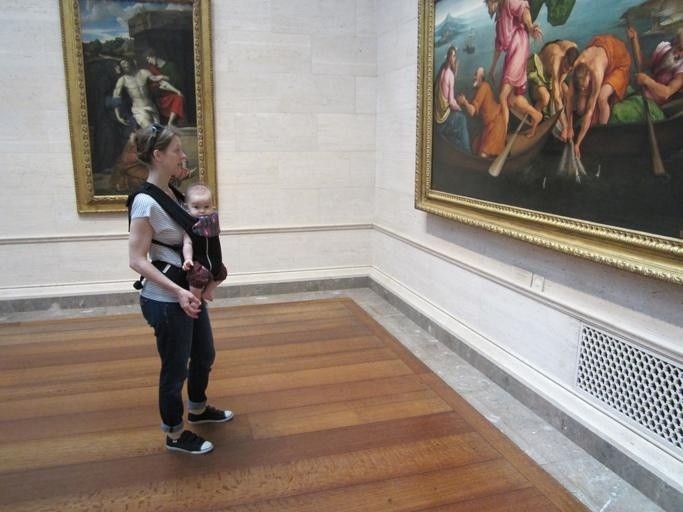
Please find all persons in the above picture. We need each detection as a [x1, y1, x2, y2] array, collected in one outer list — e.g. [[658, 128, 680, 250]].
[[126, 124, 234, 454], [429, 0, 683, 163], [94, 46, 199, 186], [181, 185, 227, 309]]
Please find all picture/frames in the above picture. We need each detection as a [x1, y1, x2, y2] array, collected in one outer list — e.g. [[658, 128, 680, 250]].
[[413, 0, 683, 287], [58, 0, 218, 215]]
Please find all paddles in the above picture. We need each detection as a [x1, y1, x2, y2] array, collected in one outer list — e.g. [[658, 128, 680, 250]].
[[632, 33, 664, 176], [489, 111, 529, 177]]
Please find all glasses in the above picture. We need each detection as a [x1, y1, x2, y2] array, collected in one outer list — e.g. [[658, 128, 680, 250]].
[[149, 122, 165, 150]]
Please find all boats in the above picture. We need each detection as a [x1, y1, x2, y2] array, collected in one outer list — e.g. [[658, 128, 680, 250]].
[[429, 102, 566, 180], [566, 92, 682, 130]]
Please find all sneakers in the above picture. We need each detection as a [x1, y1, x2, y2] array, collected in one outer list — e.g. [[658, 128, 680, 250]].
[[165, 430, 215, 455], [187, 404, 233, 425]]
